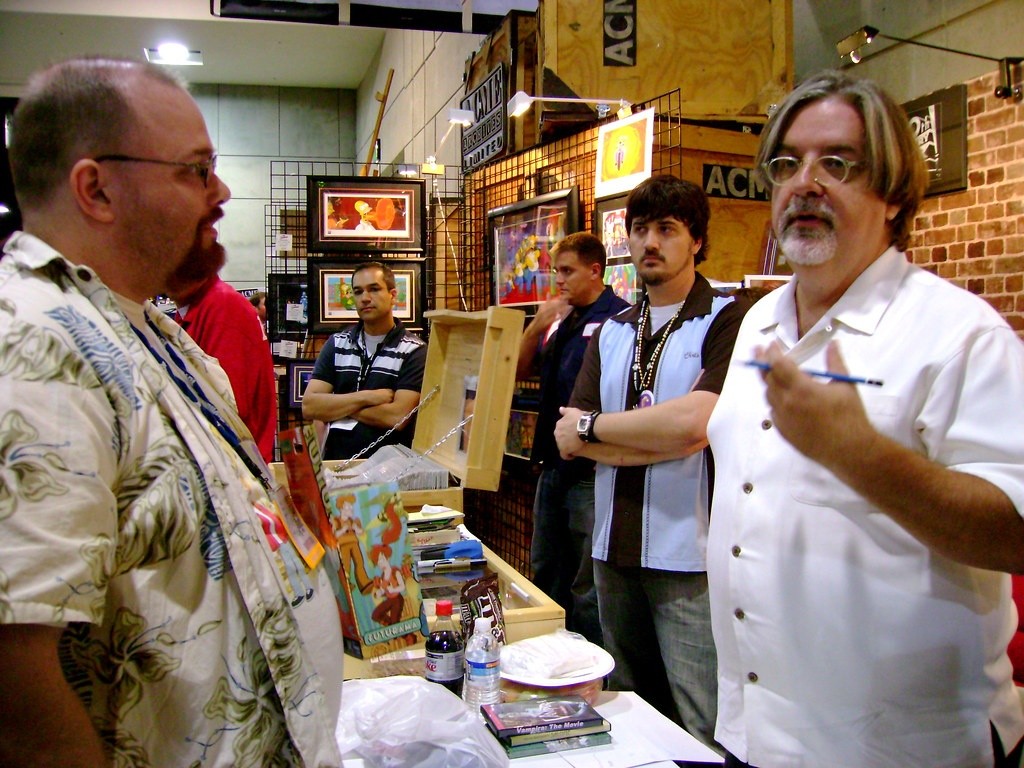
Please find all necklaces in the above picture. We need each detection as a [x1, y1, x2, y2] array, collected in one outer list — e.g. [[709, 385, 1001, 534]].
[[633, 297, 687, 409]]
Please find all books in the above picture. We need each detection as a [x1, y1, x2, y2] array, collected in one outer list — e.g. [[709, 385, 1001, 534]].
[[480, 695, 613, 759]]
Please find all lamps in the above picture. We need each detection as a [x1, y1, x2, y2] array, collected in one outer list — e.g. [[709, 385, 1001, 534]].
[[422, 107, 474, 174], [835, 25, 1024, 103], [508, 90, 633, 120]]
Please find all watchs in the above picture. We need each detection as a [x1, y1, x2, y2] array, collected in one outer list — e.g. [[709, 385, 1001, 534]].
[[576, 409, 602, 443]]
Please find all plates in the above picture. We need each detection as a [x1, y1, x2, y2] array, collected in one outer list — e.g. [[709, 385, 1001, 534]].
[[499, 640, 615, 687]]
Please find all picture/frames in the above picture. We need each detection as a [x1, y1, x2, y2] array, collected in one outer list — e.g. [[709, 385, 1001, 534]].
[[268, 273, 310, 344], [306, 257, 427, 336], [593, 188, 646, 308], [286, 358, 319, 414], [488, 185, 580, 330], [305, 175, 427, 254]]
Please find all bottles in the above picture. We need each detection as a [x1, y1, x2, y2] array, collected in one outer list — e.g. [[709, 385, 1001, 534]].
[[425, 600, 465, 701], [465, 616, 501, 724]]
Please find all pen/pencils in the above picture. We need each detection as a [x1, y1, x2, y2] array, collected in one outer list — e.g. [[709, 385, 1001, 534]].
[[737, 360, 884, 388]]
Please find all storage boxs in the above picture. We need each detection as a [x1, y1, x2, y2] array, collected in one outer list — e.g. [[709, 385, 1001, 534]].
[[422, 538, 566, 645], [321, 308, 526, 527]]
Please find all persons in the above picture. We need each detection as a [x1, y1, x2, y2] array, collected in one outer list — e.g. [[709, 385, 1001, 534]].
[[302, 261, 427, 461], [163, 273, 274, 464], [0, 53, 345, 768], [517, 232, 633, 650], [554, 172, 744, 768], [706, 70, 1024, 768], [250, 292, 266, 322]]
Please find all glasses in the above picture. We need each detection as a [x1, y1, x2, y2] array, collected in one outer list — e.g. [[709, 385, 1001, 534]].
[[766, 155, 866, 188], [94, 155, 217, 187]]
